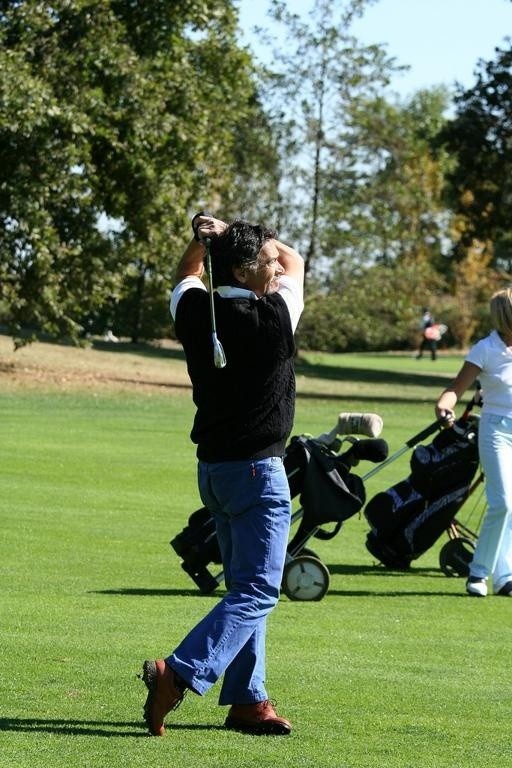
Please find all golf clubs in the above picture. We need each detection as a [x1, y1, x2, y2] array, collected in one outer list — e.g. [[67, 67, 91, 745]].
[[199, 212, 225, 366], [312, 413, 388, 468]]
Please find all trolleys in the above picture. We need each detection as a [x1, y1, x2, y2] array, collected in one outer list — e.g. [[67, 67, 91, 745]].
[[171, 407, 459, 606], [360, 391, 489, 581]]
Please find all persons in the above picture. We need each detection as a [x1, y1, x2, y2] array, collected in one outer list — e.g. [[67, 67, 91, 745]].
[[434, 288, 512, 597], [144, 212, 308, 737], [416, 308, 437, 360]]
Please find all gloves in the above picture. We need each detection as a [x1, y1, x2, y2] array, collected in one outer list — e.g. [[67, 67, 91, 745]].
[[192, 211, 209, 242]]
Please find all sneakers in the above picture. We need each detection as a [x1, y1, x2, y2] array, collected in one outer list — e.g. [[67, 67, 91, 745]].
[[224, 697, 292, 735], [466, 574, 488, 596], [498, 580, 512, 595], [143, 660, 187, 735]]
